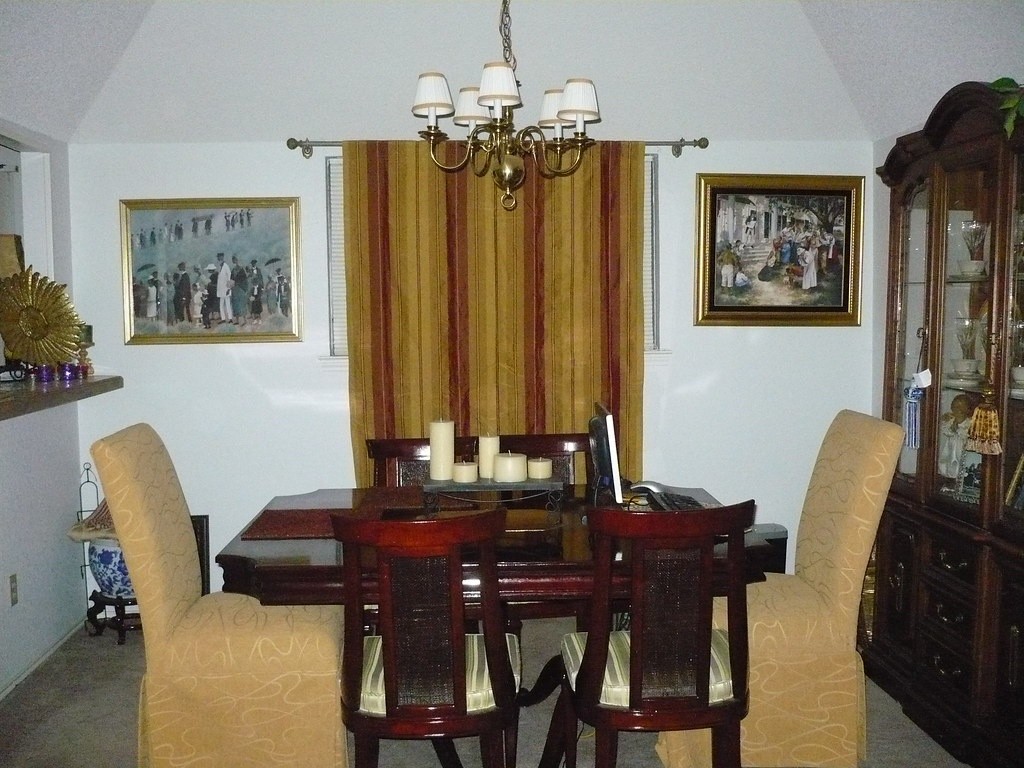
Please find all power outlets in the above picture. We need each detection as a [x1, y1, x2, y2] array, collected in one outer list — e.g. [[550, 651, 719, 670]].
[[10, 574, 18, 605]]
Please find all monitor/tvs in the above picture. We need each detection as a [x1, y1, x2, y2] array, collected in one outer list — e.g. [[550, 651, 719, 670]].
[[589, 401, 623, 508]]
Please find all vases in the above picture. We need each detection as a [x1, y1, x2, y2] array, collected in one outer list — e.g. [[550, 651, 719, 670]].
[[89, 538, 135, 598]]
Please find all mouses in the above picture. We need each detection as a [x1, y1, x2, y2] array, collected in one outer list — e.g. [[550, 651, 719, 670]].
[[630, 480, 665, 493]]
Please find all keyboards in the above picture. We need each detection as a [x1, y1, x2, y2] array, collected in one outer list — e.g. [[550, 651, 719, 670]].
[[646, 492, 705, 512]]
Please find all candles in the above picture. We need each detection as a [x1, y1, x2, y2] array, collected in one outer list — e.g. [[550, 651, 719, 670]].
[[528, 456, 552, 479], [429, 418, 454, 480], [454, 460, 478, 483], [478, 431, 499, 478], [494, 450, 527, 483], [80, 325, 93, 342]]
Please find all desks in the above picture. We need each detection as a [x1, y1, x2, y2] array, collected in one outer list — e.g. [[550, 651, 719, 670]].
[[214, 477, 770, 709]]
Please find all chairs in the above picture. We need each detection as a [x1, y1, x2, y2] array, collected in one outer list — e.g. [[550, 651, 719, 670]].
[[89, 422, 345, 767], [541, 499, 758, 767], [328, 509, 523, 767], [365, 436, 480, 634], [653, 409, 906, 767], [499, 431, 594, 634]]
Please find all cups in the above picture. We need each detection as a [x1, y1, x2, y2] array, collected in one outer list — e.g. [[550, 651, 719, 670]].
[[957, 261, 987, 275], [950, 359, 981, 377], [1012, 367, 1024, 384], [38, 365, 53, 382], [59, 362, 75, 380]]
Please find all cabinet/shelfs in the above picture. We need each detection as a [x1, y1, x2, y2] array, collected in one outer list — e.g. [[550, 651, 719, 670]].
[[856, 81, 1024, 768]]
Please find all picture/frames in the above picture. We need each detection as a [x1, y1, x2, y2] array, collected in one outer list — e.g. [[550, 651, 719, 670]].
[[692, 172, 866, 326], [118, 197, 304, 345]]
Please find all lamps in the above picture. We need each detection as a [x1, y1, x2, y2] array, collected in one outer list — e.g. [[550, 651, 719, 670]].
[[411, 1, 600, 209]]
